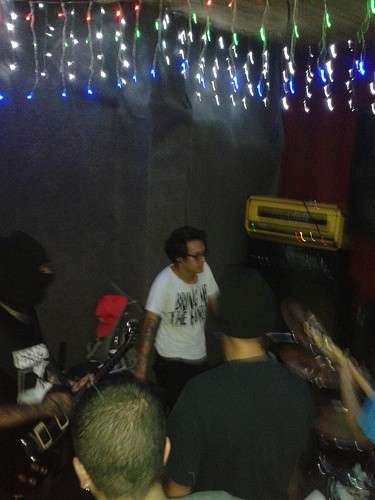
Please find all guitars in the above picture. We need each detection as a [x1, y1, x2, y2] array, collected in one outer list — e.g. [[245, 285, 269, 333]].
[[304, 316, 375, 405], [19, 318, 140, 454]]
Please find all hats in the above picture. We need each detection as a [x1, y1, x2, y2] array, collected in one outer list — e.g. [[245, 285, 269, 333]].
[[204, 269, 278, 339], [94, 293, 127, 338]]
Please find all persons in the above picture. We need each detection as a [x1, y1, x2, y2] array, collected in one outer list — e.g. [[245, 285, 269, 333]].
[[71, 270, 375, 500], [0, 228, 95, 468], [134, 225, 220, 413]]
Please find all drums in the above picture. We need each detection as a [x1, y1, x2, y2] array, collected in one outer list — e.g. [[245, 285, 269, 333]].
[[313, 406, 375, 486]]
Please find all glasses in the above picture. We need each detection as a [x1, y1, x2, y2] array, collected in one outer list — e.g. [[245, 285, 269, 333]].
[[179, 249, 211, 259]]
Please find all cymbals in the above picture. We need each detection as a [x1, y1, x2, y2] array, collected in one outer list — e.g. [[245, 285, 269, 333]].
[[278, 297, 341, 389]]
